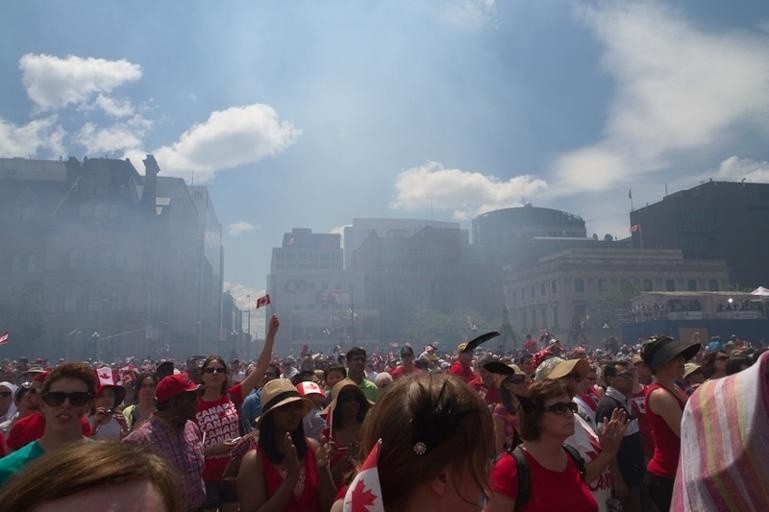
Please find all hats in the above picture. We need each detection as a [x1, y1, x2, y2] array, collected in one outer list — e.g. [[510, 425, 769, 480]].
[[482, 361, 529, 389], [0, 380, 15, 396], [254, 376, 314, 424], [318, 378, 376, 417], [23, 366, 48, 375], [91, 366, 126, 410], [645, 336, 702, 376], [296, 381, 326, 400], [456, 331, 502, 353], [154, 373, 204, 405], [533, 356, 591, 386]]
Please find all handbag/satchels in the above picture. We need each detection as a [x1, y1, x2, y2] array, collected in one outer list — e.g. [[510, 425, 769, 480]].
[[224, 389, 253, 437]]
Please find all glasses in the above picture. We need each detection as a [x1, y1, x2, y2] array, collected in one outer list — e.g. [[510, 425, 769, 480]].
[[712, 355, 730, 362], [504, 377, 526, 385], [540, 401, 579, 416], [203, 367, 227, 374], [41, 391, 95, 409], [560, 372, 583, 383], [0, 391, 12, 399], [614, 372, 634, 380]]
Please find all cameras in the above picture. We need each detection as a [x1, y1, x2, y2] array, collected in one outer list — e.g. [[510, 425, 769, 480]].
[[103, 406, 114, 417], [231, 436, 242, 443]]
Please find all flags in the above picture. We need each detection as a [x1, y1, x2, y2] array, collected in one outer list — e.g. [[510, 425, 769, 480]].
[[255, 294, 271, 308]]
